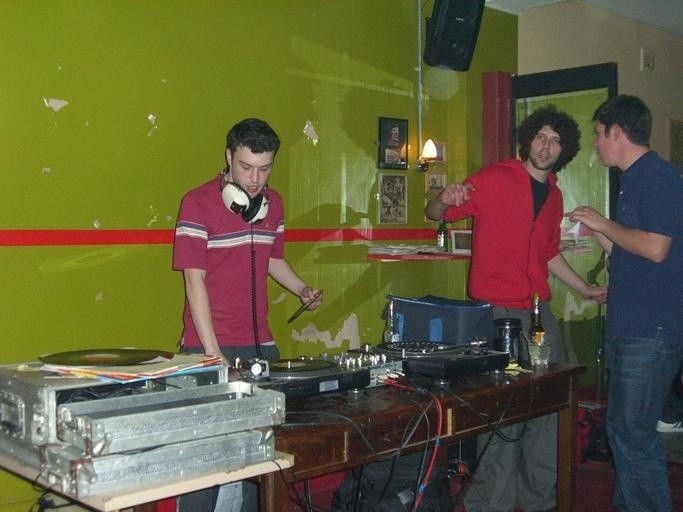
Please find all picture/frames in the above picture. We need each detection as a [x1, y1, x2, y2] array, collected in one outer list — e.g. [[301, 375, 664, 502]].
[[377, 115, 447, 225], [451, 230, 473, 255]]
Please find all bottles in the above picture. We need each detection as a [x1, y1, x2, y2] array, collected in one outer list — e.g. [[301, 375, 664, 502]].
[[528, 292, 546, 365], [437, 219, 448, 253]]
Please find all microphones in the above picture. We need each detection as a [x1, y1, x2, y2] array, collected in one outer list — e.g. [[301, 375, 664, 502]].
[[262, 200, 272, 207]]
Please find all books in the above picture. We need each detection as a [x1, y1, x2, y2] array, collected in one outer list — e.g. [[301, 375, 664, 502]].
[[367, 242, 441, 255]]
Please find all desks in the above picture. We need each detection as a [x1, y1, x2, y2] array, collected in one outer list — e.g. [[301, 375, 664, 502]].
[[0, 449, 294, 512], [247, 361, 587, 510]]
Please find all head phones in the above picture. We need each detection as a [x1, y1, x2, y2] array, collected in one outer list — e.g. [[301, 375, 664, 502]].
[[219, 167, 271, 226]]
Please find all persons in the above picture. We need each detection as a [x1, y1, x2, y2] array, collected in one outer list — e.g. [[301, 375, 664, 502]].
[[174, 118, 322, 512], [562, 93, 682, 511], [654, 360, 682, 437], [424, 103, 607, 511]]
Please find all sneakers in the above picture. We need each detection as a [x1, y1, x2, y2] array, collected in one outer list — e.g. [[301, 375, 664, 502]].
[[656, 420, 683, 432]]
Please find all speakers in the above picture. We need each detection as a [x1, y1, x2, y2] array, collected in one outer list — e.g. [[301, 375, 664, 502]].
[[422, 0, 485, 72]]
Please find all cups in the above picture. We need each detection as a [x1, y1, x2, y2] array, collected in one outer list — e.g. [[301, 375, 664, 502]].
[[494, 318, 524, 366]]
